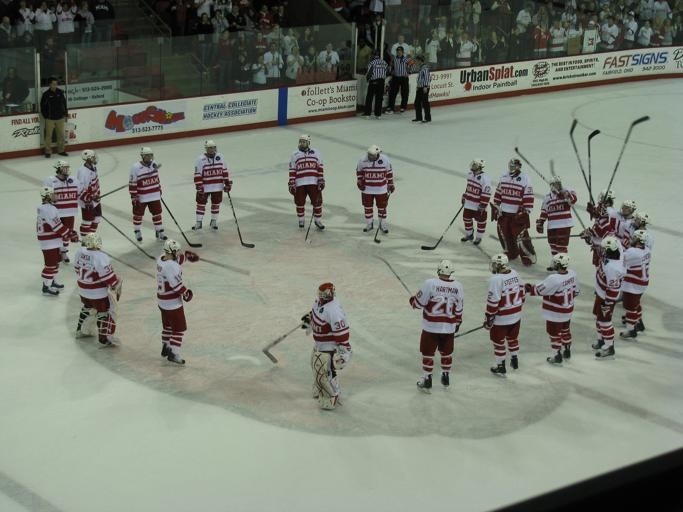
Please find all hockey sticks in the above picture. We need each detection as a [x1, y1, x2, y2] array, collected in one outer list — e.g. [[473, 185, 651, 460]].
[[489, 231, 582, 241], [549, 161, 586, 228], [160, 197, 202, 248], [227, 192, 255, 248], [97, 164, 162, 198], [514, 146, 559, 195], [604, 116, 650, 201], [374, 255, 413, 296], [305, 190, 321, 246], [101, 215, 157, 260], [262, 322, 303, 363], [373, 191, 390, 243], [569, 119, 600, 206], [421, 206, 463, 250]]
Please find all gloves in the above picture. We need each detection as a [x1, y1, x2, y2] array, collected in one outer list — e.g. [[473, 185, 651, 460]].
[[300, 310, 315, 329], [387, 184, 395, 193], [131, 196, 142, 208], [317, 179, 325, 192], [196, 184, 204, 193], [356, 180, 366, 192], [524, 283, 533, 293], [223, 183, 232, 192], [461, 197, 466, 204], [483, 312, 497, 331], [287, 181, 297, 196], [478, 206, 485, 216], [490, 202, 498, 222], [67, 230, 79, 243], [185, 250, 199, 263], [600, 303, 612, 318], [535, 219, 545, 235], [182, 288, 193, 303]]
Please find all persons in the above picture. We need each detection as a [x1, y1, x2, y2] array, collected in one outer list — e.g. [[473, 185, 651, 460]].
[[355, 145, 396, 235], [76, 149, 103, 240], [39, 79, 70, 158], [299, 282, 352, 407], [358, 51, 391, 119], [154, 239, 192, 362], [410, 260, 462, 391], [73, 231, 121, 347], [287, 135, 325, 231], [2, 0, 683, 90], [1, 67, 31, 108], [190, 141, 231, 232], [34, 186, 71, 294], [128, 147, 169, 242], [462, 159, 654, 375], [411, 55, 432, 123], [384, 47, 410, 113], [42, 158, 80, 263]]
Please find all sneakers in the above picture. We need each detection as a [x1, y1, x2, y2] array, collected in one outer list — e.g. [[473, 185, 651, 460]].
[[191, 221, 202, 231], [156, 229, 168, 240], [363, 221, 374, 233], [61, 253, 70, 263], [209, 219, 219, 230], [135, 230, 142, 242], [167, 352, 185, 364], [51, 279, 65, 288], [298, 220, 305, 228], [413, 319, 644, 389], [96, 339, 118, 349], [44, 153, 51, 159], [460, 233, 474, 242], [161, 342, 172, 357], [315, 220, 325, 229], [360, 106, 430, 123], [74, 330, 93, 339], [57, 151, 69, 157], [42, 281, 59, 295], [380, 221, 389, 233], [472, 237, 481, 246]]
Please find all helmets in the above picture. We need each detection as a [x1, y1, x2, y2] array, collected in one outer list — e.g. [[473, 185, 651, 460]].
[[204, 139, 217, 148], [139, 146, 154, 155], [81, 149, 95, 161], [472, 158, 486, 169], [319, 282, 335, 303], [549, 174, 651, 270], [162, 239, 182, 254], [437, 259, 456, 277], [53, 160, 70, 170], [39, 186, 54, 197], [508, 158, 522, 176], [490, 252, 509, 265], [367, 144, 382, 156], [299, 134, 311, 142], [81, 232, 103, 250]]
